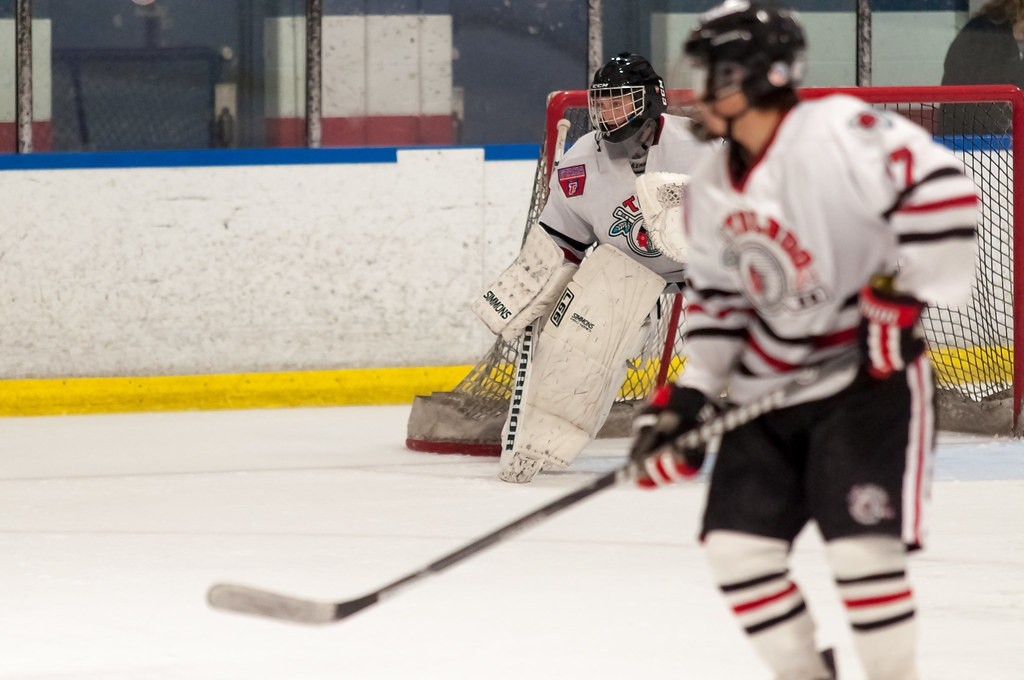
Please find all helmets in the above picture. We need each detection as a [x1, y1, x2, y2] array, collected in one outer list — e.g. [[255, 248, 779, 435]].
[[683, 0, 809, 111], [590, 52, 667, 144]]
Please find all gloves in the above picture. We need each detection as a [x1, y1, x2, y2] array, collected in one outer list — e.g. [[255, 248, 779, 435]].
[[856, 281, 928, 379], [623, 386, 715, 489]]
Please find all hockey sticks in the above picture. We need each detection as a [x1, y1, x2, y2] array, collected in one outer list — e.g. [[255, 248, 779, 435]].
[[204, 332, 929, 629], [497, 117, 572, 485]]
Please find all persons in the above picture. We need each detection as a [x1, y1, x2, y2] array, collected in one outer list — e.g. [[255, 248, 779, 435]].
[[940, 0, 1024, 135], [470, 52, 729, 484], [630, 0, 984, 680]]
